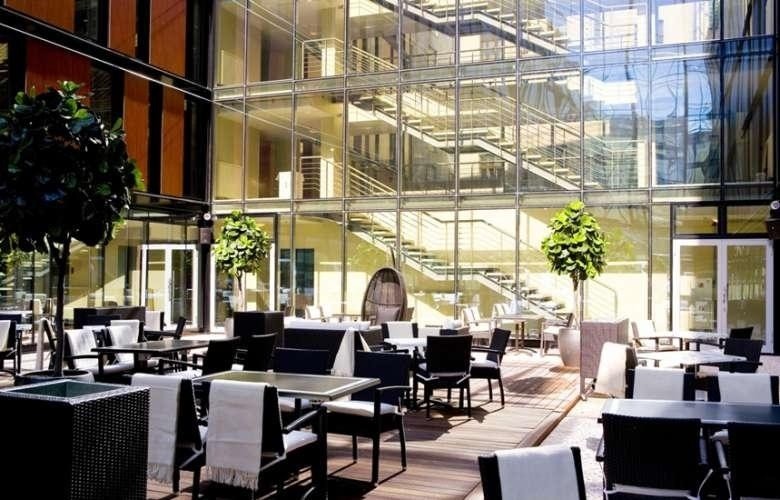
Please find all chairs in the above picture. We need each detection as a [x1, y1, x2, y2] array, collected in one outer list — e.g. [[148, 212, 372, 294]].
[[594, 321, 780, 500], [24, 295, 510, 418], [313, 348, 411, 486], [541, 311, 574, 354], [128, 373, 208, 499], [204, 379, 317, 499], [478, 444, 587, 500]]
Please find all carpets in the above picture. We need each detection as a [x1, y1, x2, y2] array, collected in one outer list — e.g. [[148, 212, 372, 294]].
[[146, 362, 591, 500]]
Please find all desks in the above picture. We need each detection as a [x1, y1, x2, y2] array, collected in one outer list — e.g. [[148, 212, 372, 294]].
[[189, 369, 380, 500], [579, 317, 629, 401]]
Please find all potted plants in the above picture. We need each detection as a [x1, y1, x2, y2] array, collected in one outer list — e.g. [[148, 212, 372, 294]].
[[541, 200, 607, 366], [211, 211, 272, 337], [0, 81, 145, 386]]
